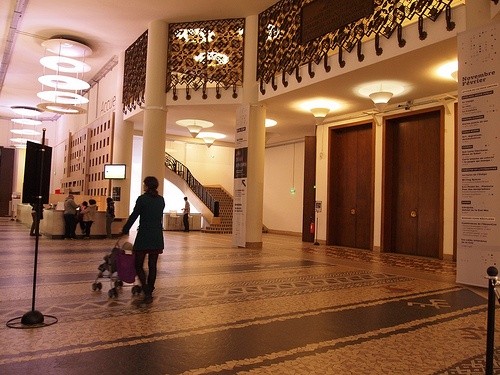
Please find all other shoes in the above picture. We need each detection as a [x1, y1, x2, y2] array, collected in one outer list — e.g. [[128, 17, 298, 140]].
[[144, 285, 155, 303]]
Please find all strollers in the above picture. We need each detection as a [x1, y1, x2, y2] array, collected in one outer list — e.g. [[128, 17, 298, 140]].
[[92, 233, 141, 298]]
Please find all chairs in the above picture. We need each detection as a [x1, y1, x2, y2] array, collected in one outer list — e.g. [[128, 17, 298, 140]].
[[168, 210, 180, 231], [183, 209, 194, 231]]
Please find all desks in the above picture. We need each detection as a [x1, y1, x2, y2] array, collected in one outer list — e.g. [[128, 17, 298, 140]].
[[163, 212, 202, 231]]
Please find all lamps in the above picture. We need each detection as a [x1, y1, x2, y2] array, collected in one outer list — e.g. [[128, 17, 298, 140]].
[[368, 84, 393, 112], [8, 106, 43, 149], [451, 70, 458, 82], [203, 136, 216, 149], [310, 108, 330, 124], [35, 35, 93, 115], [186, 120, 203, 138]]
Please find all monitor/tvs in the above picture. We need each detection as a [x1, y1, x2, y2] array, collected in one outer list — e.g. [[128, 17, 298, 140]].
[[104, 164, 126, 180]]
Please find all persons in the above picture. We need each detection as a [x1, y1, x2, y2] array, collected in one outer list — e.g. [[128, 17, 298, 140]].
[[78, 199, 98, 240], [181, 197, 190, 232], [63, 194, 80, 240], [30, 192, 43, 237], [105, 197, 115, 240], [118, 176, 165, 306]]
